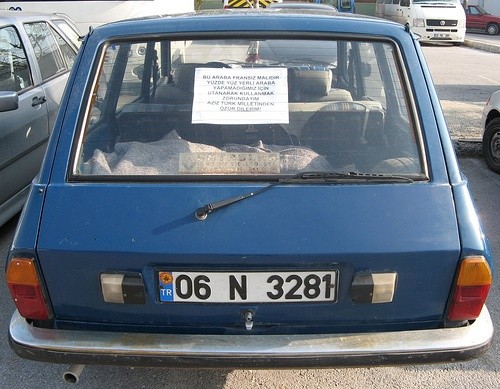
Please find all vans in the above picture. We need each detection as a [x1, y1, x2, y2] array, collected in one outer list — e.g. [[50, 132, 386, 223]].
[[375, 0, 467, 46]]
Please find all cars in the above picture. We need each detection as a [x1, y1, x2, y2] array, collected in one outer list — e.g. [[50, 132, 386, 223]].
[[1, 10, 107, 230], [481, 90, 500, 174], [462, 4, 500, 36], [248, 2, 374, 89], [4, 5, 493, 387]]
